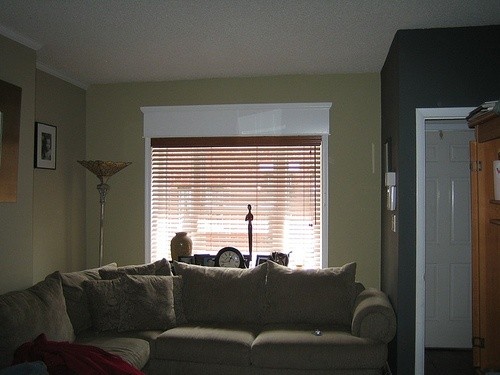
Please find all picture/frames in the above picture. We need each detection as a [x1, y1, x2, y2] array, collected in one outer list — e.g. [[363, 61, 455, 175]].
[[0, 80, 21, 203], [34, 122, 57, 169], [194, 253, 216, 267], [179, 256, 194, 264], [385, 139, 391, 172], [255, 255, 272, 267]]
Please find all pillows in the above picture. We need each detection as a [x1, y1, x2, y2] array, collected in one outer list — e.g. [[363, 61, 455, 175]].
[[173, 259, 266, 324], [117, 271, 177, 334], [83, 275, 188, 332], [0, 271, 76, 369], [261, 260, 357, 330], [98, 258, 174, 279], [60, 263, 117, 336]]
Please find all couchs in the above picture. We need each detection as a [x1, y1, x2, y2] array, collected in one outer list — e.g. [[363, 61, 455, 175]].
[[0, 282, 397, 375]]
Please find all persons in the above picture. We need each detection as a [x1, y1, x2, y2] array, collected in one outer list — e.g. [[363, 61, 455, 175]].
[[42, 138, 51, 160]]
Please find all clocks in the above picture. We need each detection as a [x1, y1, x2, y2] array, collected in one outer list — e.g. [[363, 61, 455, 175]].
[[215, 247, 244, 268]]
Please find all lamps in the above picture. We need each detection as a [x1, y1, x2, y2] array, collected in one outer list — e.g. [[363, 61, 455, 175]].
[[76, 160, 132, 267]]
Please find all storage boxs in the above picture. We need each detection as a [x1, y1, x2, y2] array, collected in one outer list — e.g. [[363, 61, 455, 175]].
[[469, 113, 499, 143]]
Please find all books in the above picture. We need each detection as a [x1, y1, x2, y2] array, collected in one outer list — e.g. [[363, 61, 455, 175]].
[[465, 100, 499, 122]]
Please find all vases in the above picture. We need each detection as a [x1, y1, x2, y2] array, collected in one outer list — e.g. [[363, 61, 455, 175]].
[[171, 232, 193, 262]]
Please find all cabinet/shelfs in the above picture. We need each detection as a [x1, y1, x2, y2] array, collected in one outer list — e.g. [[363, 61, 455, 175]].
[[476, 139, 500, 374]]
